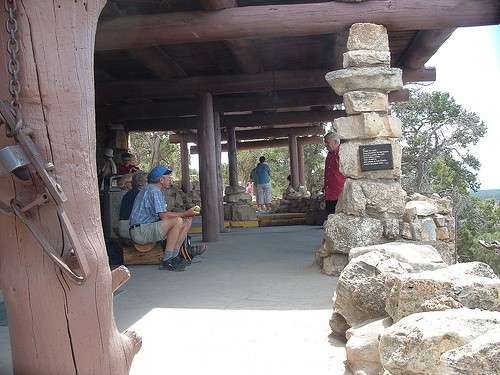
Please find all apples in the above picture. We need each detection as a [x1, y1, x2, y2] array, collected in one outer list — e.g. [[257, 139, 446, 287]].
[[194, 206, 201, 211]]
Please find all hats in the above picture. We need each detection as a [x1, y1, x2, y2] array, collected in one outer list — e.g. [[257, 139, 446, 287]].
[[147, 166, 172, 183], [103, 147, 114, 157], [121, 153, 131, 160]]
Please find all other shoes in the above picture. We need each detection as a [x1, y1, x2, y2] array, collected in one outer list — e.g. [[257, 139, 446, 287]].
[[193, 245, 206, 256], [258, 209, 274, 214]]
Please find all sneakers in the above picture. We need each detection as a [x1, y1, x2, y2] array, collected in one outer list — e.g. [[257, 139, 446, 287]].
[[160, 255, 190, 271]]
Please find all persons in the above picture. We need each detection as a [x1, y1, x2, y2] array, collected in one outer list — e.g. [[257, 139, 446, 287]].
[[246, 182, 253, 195], [287, 175, 291, 185], [256, 156, 271, 212], [118, 171, 207, 259], [128, 165, 200, 272], [250, 163, 265, 211], [322, 132, 347, 220], [97, 147, 140, 228]]
